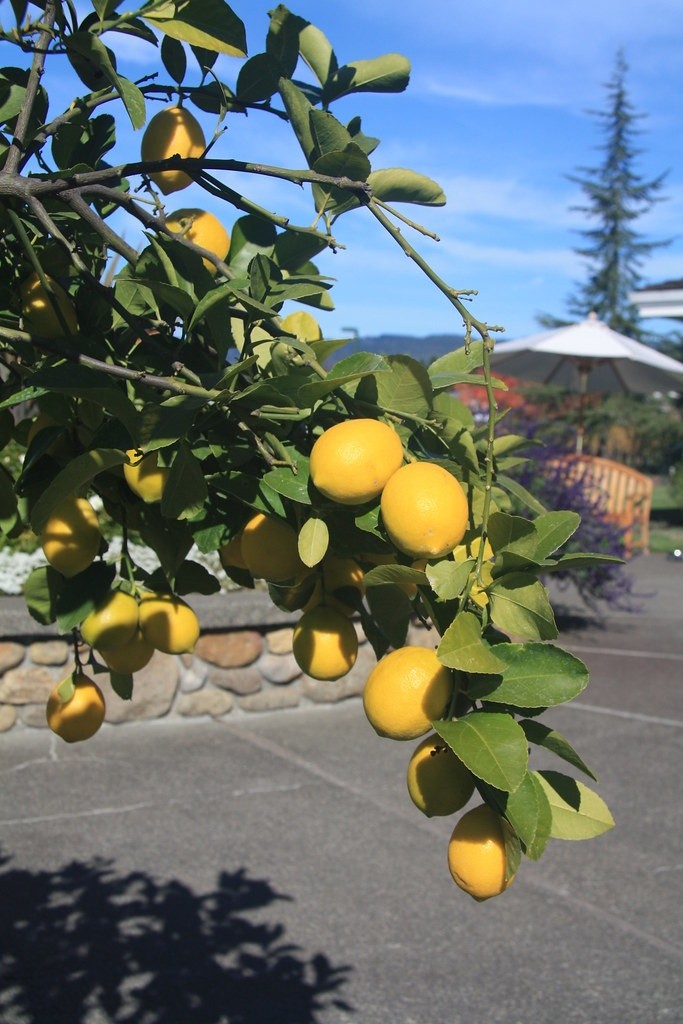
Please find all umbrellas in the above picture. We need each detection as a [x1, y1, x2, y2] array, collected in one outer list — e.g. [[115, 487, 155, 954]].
[[489, 312, 683, 455]]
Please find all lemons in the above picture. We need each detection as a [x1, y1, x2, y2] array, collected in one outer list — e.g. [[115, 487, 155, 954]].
[[42, 105, 521, 902]]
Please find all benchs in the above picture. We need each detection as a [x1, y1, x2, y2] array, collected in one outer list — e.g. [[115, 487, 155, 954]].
[[543, 454, 654, 558]]
